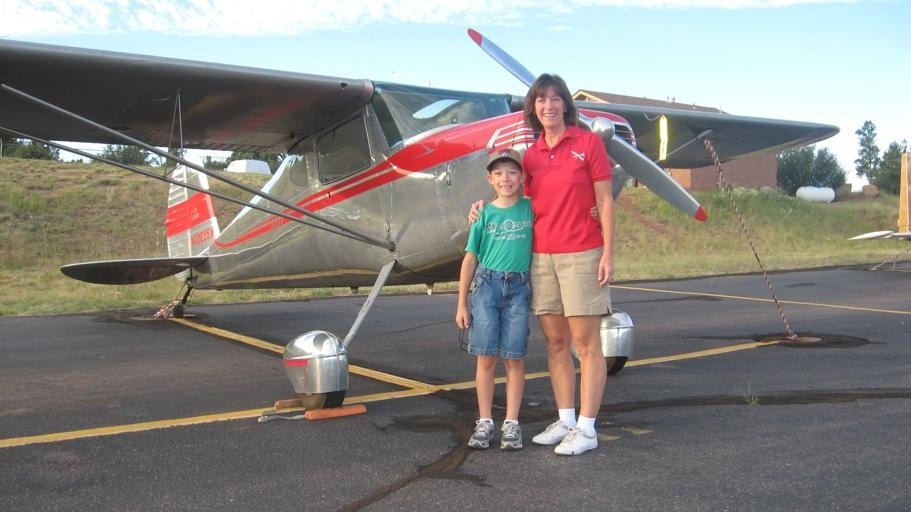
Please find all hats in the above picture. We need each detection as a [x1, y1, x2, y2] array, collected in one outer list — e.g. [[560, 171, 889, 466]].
[[486, 149, 523, 173]]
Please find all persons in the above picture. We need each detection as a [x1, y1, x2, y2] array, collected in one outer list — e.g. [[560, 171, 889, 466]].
[[467, 72, 618, 457], [451, 146, 539, 452]]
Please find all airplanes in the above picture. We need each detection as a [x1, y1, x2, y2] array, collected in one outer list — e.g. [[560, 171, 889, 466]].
[[1, 26, 845, 416], [847, 150, 911, 270]]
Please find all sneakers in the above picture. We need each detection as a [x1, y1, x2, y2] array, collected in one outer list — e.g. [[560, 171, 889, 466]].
[[531, 419, 574, 445], [468, 419, 495, 450], [500, 421, 523, 451], [554, 426, 598, 456]]
